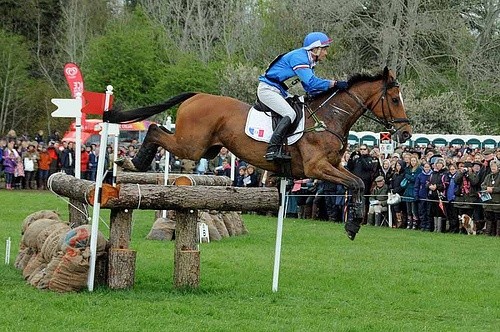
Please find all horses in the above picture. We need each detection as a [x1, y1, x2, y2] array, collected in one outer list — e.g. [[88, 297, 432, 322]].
[[104, 66, 413, 240]]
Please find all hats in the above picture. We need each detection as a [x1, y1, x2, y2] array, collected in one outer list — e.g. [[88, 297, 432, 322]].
[[375, 176, 384, 181], [490, 160, 500, 168], [466, 147, 472, 153], [456, 160, 466, 166], [484, 151, 491, 155], [472, 161, 483, 168], [27, 143, 35, 149], [391, 153, 402, 160], [434, 158, 446, 169]]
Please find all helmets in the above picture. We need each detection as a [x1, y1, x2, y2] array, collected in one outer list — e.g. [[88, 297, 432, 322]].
[[302, 32, 333, 50]]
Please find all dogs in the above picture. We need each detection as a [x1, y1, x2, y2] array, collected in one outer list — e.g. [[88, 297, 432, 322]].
[[458, 214, 486, 236]]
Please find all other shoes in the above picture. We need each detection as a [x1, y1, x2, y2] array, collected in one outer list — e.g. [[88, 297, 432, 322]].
[[421, 228, 430, 231]]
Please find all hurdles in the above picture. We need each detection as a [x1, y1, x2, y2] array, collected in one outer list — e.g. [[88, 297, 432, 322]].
[[48, 62, 279, 289]]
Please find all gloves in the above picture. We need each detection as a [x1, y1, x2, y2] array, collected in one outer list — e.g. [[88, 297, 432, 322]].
[[334, 80, 348, 91], [415, 196, 419, 200], [370, 199, 381, 204]]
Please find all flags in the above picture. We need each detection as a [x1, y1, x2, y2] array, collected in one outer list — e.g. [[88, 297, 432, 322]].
[[63, 62, 86, 128]]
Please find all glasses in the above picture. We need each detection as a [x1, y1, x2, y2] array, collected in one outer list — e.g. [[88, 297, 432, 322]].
[[360, 148, 366, 150]]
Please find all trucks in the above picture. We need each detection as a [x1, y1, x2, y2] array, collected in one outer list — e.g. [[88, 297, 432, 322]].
[[61, 118, 161, 150]]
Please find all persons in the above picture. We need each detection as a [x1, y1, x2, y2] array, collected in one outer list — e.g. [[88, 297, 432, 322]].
[[256, 32, 348, 161], [0, 129, 500, 238]]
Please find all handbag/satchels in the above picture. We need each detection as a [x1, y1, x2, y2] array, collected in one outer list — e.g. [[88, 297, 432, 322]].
[[400, 178, 408, 187], [387, 192, 401, 205], [24, 157, 35, 171]]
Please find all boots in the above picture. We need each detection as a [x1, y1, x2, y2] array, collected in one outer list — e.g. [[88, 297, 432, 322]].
[[406, 215, 412, 229], [5, 183, 11, 189], [434, 217, 442, 232], [485, 220, 493, 235], [364, 213, 374, 226], [15, 183, 22, 190], [395, 210, 405, 228], [496, 220, 500, 236], [374, 212, 382, 227], [413, 215, 419, 229], [266, 116, 293, 162]]
